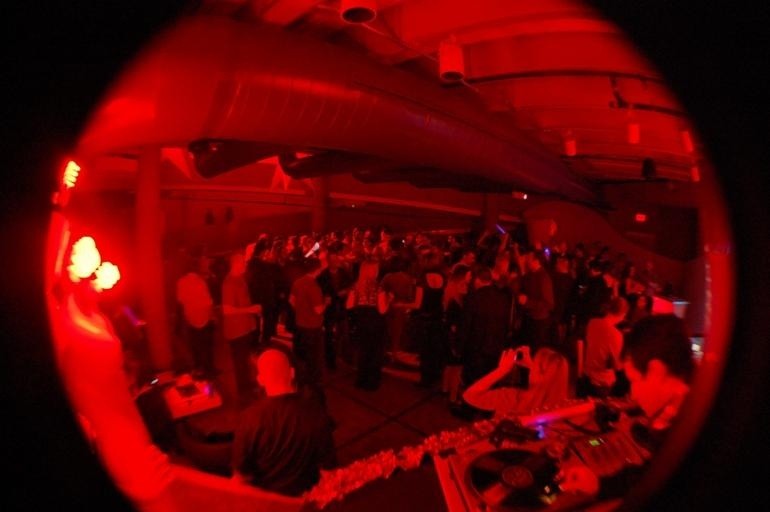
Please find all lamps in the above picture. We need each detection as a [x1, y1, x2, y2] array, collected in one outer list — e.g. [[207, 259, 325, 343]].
[[436, 39, 467, 83], [566, 123, 702, 183], [339, 0, 378, 23]]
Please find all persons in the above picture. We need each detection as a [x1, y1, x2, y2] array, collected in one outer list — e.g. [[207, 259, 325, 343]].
[[224, 345, 338, 497], [171, 222, 701, 435]]
